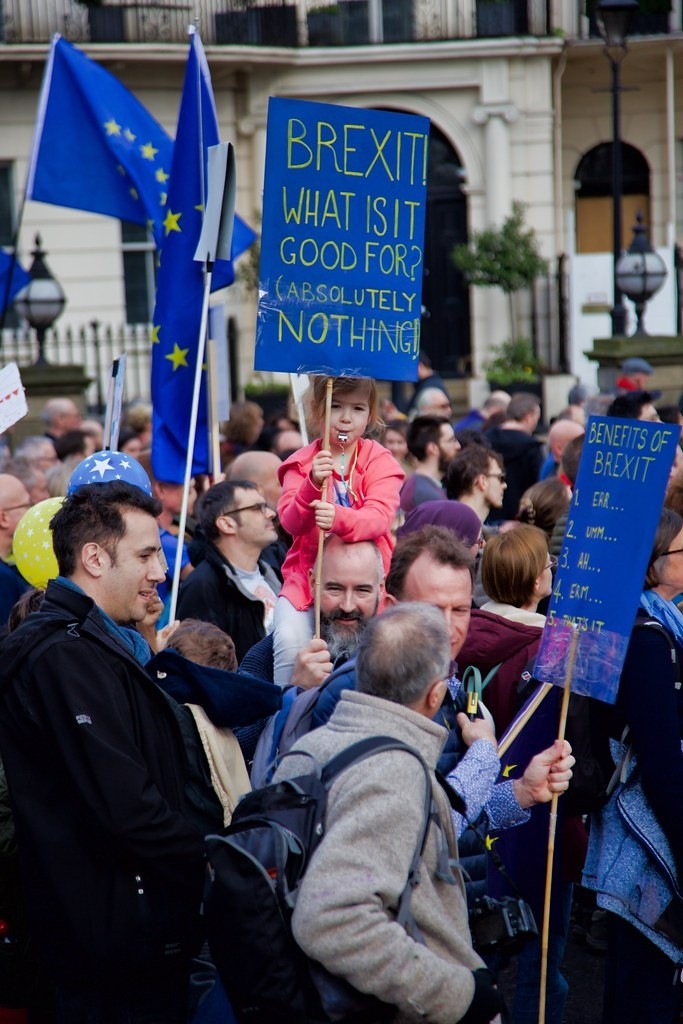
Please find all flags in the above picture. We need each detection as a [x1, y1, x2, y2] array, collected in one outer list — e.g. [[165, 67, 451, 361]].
[[28, 40, 259, 260], [150, 36, 222, 483], [482, 687, 563, 900]]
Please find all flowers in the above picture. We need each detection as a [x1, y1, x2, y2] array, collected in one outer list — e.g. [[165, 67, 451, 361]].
[[479, 337, 547, 386]]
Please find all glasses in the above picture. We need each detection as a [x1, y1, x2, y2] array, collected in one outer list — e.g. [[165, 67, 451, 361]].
[[223, 502, 271, 516], [544, 555, 558, 570], [473, 533, 484, 548], [475, 472, 506, 486], [2, 499, 34, 511]]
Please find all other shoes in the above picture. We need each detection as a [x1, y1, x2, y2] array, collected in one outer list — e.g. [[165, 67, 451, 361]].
[[572, 907, 620, 943]]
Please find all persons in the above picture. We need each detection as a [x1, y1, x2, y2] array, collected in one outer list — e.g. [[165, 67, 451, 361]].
[[1, 356, 682, 1024], [272, 602, 508, 1024]]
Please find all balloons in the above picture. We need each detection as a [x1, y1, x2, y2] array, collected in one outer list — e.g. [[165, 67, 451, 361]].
[[12, 496, 67, 592], [68, 449, 152, 497]]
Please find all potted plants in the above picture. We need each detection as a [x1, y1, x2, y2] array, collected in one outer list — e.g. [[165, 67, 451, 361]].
[[451, 197, 551, 430], [239, 238, 292, 427]]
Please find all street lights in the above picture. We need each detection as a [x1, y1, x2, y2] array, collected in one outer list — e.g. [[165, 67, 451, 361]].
[[574, 0, 655, 338]]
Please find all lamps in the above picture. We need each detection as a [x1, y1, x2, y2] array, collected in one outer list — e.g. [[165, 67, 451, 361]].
[[15, 229, 68, 365], [618, 208, 669, 340]]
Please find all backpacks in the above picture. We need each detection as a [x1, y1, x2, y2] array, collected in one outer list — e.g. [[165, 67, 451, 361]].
[[520, 616, 681, 817], [201, 736, 432, 1024]]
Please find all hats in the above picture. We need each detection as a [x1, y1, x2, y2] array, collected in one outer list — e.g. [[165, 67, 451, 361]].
[[623, 358, 654, 377]]
